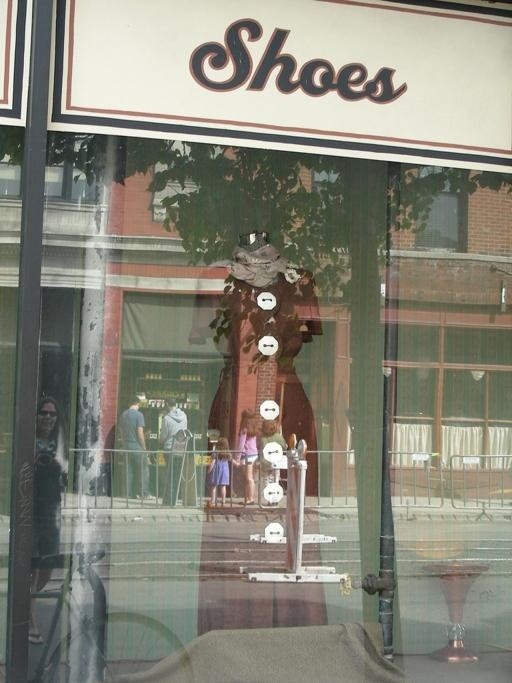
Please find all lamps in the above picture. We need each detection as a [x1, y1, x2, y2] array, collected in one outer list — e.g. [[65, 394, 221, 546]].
[[383, 366, 392, 379], [470, 368, 487, 383]]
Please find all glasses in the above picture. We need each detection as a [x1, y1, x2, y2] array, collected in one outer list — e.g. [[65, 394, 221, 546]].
[[38, 409, 57, 416]]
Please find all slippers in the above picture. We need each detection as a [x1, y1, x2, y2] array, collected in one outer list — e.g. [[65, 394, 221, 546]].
[[27, 632, 43, 644]]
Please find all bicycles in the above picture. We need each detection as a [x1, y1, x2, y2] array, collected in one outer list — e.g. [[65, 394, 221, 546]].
[[0, 550, 198, 683]]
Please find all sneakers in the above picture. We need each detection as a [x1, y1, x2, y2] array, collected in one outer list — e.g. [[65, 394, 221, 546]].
[[243, 500, 254, 505], [137, 494, 156, 499]]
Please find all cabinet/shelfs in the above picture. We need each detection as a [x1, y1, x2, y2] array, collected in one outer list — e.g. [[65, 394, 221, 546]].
[[130, 373, 211, 452]]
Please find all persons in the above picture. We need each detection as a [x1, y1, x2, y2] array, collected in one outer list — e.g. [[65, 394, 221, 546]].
[[204, 434, 241, 509], [254, 417, 288, 508], [25, 394, 70, 647], [153, 394, 189, 507], [232, 407, 260, 508], [114, 394, 152, 503], [186, 230, 328, 632]]
